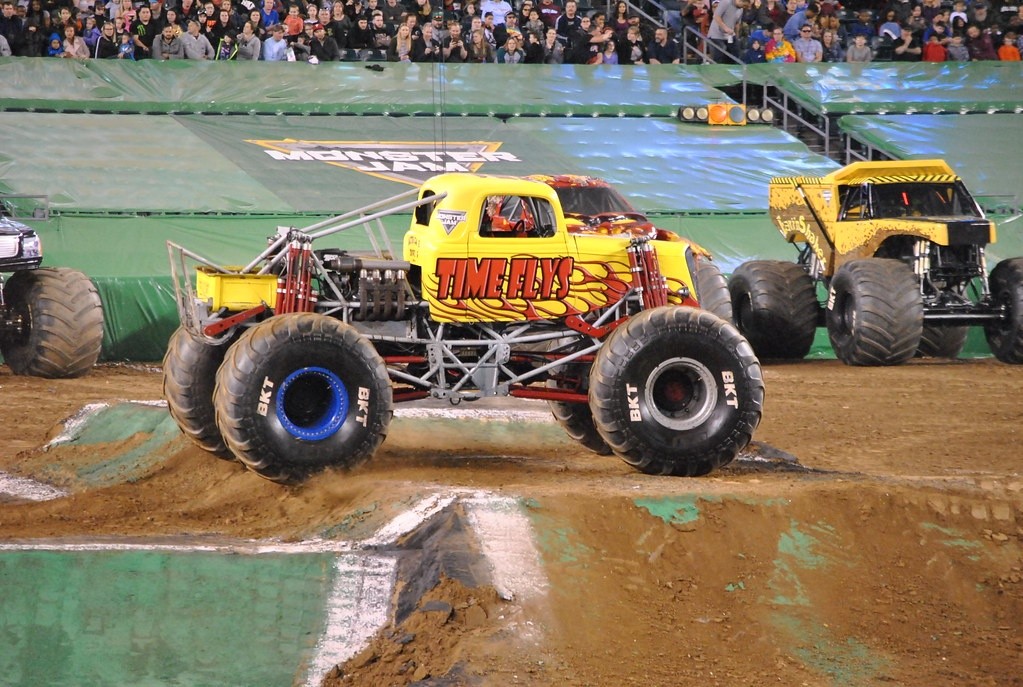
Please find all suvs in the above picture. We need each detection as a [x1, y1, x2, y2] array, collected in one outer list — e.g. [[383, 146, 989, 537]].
[[721, 159, 1023, 369], [0, 200, 107, 381], [155, 172, 766, 489]]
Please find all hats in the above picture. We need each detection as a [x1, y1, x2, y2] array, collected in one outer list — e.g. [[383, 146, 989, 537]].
[[504, 11, 517, 22], [313, 24, 323, 32]]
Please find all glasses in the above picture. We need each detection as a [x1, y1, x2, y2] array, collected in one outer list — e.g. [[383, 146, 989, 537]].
[[802, 30, 812, 33], [320, 6, 329, 11], [508, 16, 517, 20], [523, 8, 532, 11]]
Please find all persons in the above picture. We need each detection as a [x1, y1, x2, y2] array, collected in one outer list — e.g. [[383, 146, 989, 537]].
[[847, 33, 871, 62], [891, 25, 922, 62], [0, 0, 680, 64], [681, 1, 1023, 63]]
